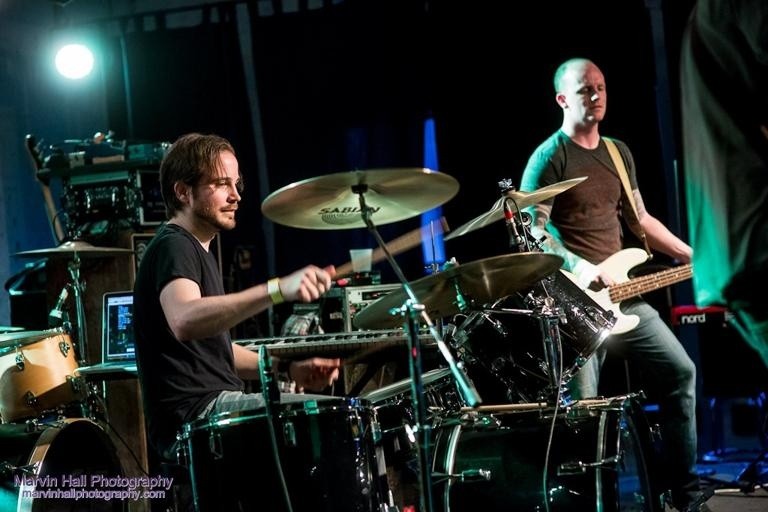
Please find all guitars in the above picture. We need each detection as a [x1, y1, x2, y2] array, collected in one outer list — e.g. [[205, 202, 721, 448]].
[[548, 244, 695, 336]]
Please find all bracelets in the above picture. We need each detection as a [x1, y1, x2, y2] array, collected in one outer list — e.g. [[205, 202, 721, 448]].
[[265, 276, 286, 306]]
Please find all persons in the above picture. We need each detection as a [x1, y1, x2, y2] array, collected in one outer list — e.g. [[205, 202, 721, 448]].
[[131, 130, 341, 483], [511, 54, 713, 512]]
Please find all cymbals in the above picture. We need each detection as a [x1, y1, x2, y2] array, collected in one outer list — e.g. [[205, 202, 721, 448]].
[[442, 175, 590, 242], [352, 251, 565, 329], [9, 239, 137, 258], [261, 167, 461, 232]]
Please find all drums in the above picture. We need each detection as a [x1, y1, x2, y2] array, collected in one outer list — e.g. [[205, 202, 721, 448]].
[[0, 325, 26, 335], [0, 415, 129, 512], [448, 246, 616, 403], [367, 366, 466, 493], [180, 397, 379, 511], [0, 326, 88, 428], [432, 394, 663, 512]]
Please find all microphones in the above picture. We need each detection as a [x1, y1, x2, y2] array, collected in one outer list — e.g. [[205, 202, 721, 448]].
[[503, 206, 526, 245], [46, 282, 73, 328]]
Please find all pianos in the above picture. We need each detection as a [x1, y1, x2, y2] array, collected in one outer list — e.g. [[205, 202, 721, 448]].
[[233, 326, 448, 362]]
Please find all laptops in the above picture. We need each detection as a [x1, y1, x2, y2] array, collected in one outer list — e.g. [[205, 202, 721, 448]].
[[77, 290, 138, 375]]
